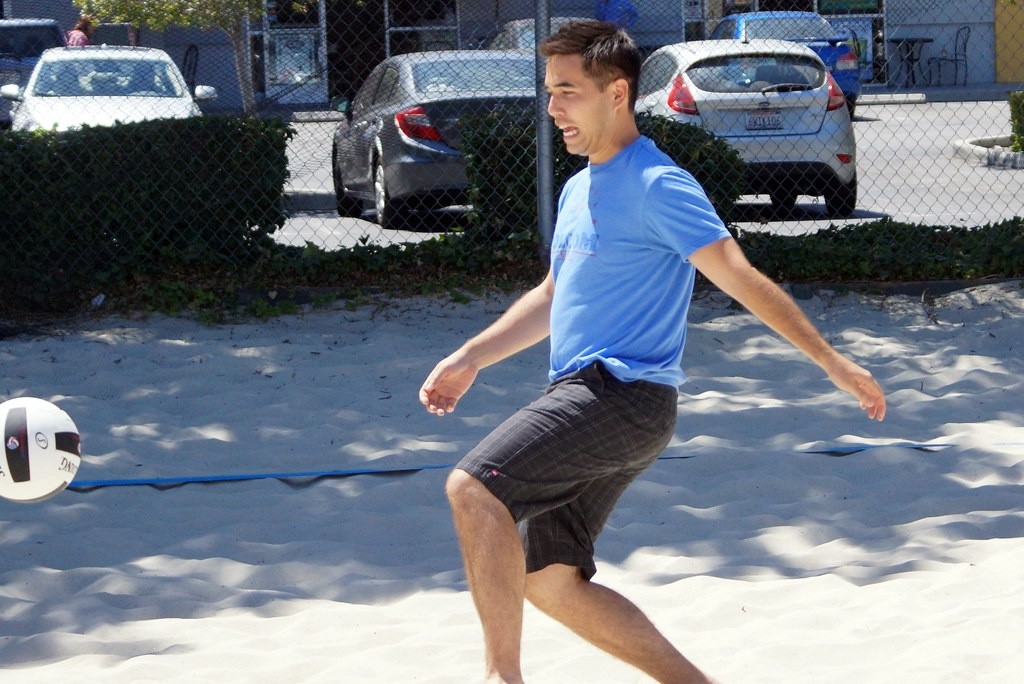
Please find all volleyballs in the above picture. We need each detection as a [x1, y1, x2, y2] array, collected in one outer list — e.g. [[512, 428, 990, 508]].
[[0, 396, 84, 504]]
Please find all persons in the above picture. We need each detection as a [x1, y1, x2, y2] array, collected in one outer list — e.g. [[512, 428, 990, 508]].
[[64, 15, 95, 46], [417, 19, 886, 684]]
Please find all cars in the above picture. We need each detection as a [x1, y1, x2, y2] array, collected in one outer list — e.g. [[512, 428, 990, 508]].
[[0, 17, 78, 126], [473, 16, 602, 58], [327, 50, 544, 229], [0, 43, 218, 144], [707, 9, 862, 120], [635, 38, 859, 216]]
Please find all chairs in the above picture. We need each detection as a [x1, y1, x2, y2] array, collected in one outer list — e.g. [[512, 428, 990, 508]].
[[428, 76, 452, 90], [757, 64, 811, 91], [124, 60, 164, 93], [512, 75, 533, 88], [928, 26, 970, 86], [50, 62, 92, 96]]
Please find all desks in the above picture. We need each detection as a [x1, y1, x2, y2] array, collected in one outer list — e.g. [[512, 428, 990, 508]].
[[886, 37, 934, 88]]
